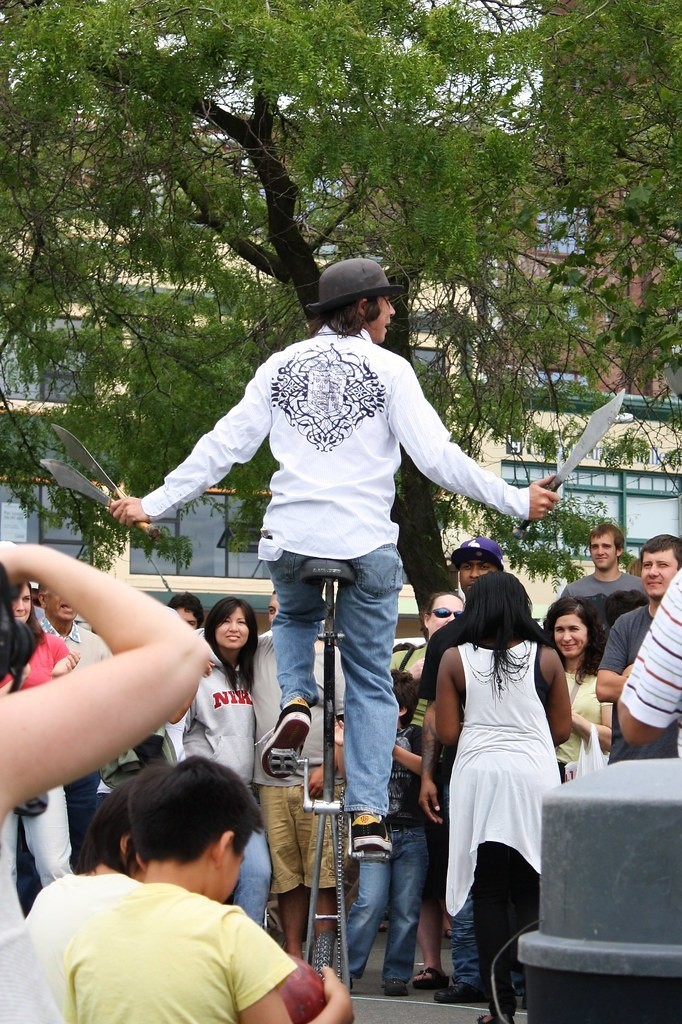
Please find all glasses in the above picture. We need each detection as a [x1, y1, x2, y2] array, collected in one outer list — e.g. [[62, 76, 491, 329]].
[[428, 608, 464, 619]]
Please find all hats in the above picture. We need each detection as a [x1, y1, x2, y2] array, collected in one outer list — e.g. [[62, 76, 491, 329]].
[[306, 258, 403, 312], [451, 537, 504, 571]]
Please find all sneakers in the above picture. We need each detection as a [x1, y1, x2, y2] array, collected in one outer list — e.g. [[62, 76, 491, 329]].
[[253, 696, 311, 779], [351, 815, 392, 852]]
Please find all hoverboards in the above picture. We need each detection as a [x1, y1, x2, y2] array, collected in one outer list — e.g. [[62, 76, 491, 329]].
[[267, 560, 355, 994]]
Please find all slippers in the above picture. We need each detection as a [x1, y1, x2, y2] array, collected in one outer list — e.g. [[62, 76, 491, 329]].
[[412, 966, 450, 991]]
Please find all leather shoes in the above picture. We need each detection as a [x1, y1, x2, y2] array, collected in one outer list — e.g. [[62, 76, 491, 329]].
[[433, 982, 488, 1003]]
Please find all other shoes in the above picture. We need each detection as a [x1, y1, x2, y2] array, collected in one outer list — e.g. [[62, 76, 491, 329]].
[[476, 1014, 515, 1024], [381, 977, 408, 996]]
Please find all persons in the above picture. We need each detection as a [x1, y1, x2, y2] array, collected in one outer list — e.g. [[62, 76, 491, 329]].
[[417, 536, 505, 1003], [594, 534, 682, 764], [16, 582, 101, 914], [542, 594, 613, 783], [202, 590, 346, 959], [63, 754, 355, 1024], [560, 523, 647, 611], [169, 597, 272, 926], [166, 592, 205, 630], [24, 775, 147, 1012], [616, 567, 682, 760], [435, 572, 572, 1024], [334, 669, 429, 996], [0, 544, 212, 1024], [390, 592, 465, 988], [111, 258, 561, 854], [0, 580, 81, 889]]
